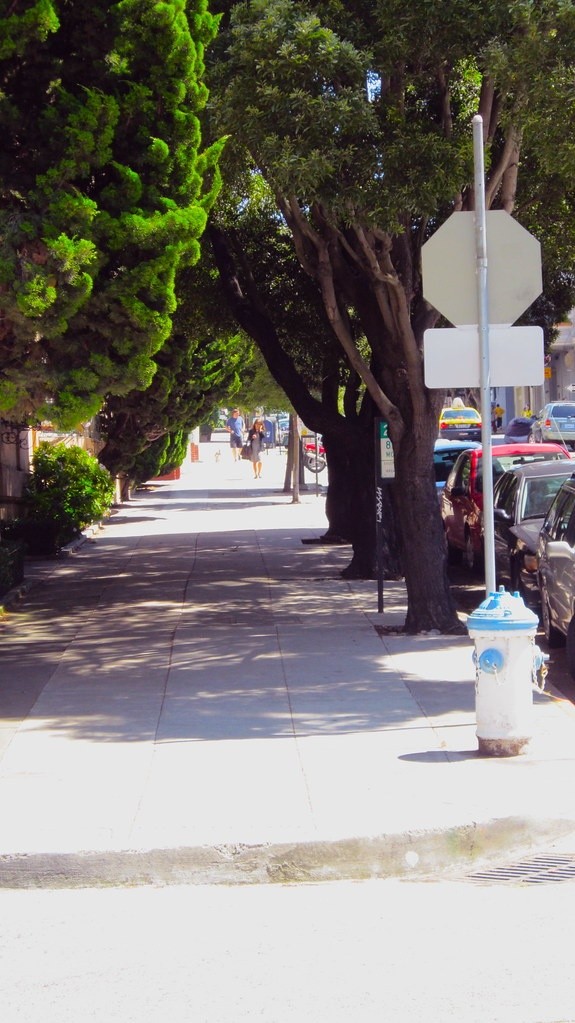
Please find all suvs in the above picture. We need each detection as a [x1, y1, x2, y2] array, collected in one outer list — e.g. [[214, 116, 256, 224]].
[[527, 401, 575, 450]]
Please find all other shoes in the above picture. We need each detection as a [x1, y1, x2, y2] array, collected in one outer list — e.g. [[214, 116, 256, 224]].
[[254, 473, 261, 479]]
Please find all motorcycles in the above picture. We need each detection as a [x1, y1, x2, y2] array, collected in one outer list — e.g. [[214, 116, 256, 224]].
[[304, 442, 328, 473]]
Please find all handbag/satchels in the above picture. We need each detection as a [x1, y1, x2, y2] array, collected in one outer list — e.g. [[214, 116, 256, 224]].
[[240, 440, 252, 459]]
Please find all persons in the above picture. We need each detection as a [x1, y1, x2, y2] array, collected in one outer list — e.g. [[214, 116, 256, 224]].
[[246, 418, 266, 479], [521, 407, 526, 417], [491, 405, 498, 434], [495, 404, 504, 430], [526, 403, 532, 418], [226, 409, 245, 462]]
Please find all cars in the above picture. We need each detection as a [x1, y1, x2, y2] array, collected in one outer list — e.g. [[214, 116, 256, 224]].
[[274, 416, 304, 446], [536, 472, 575, 675], [439, 397, 481, 442], [480, 458, 575, 594], [504, 418, 536, 445], [433, 442, 482, 494], [438, 441, 574, 570]]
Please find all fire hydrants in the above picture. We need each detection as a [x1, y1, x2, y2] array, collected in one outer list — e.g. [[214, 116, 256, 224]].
[[464, 590, 551, 756]]
